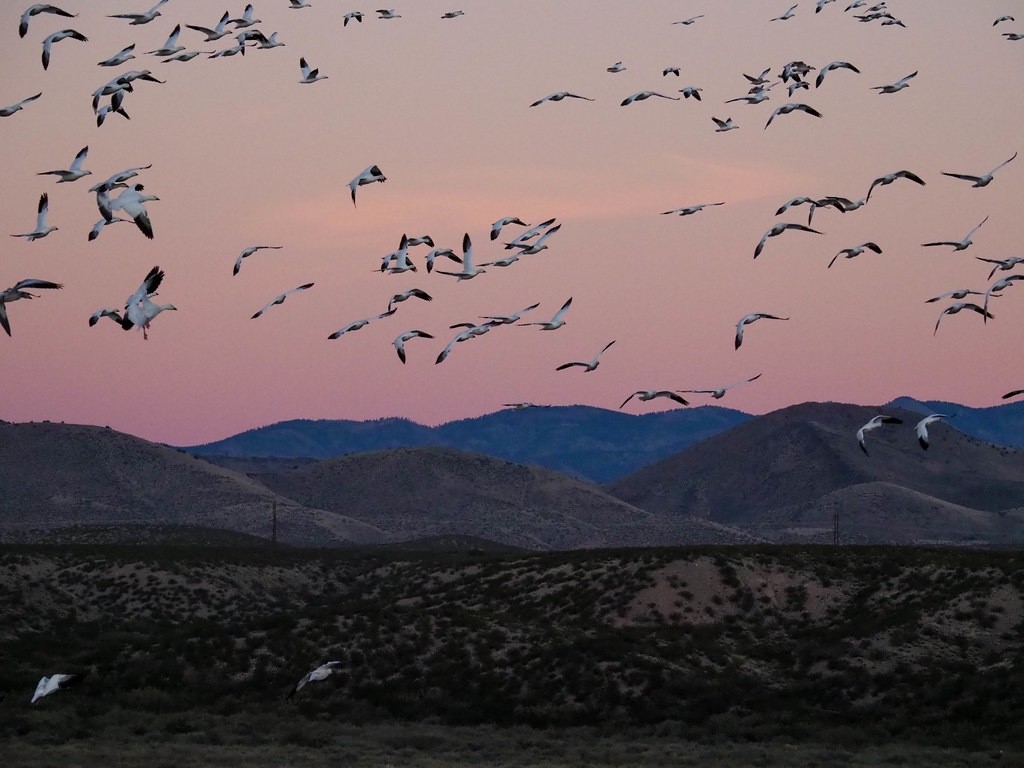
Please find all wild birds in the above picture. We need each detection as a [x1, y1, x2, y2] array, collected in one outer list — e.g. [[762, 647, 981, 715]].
[[977, 257, 1024, 281], [1001, 389, 1024, 400], [984, 274, 1024, 325], [941, 152, 1018, 189], [0, 145, 177, 339], [993, 16, 1014, 26], [922, 216, 989, 252], [914, 414, 958, 451], [933, 303, 994, 334], [866, 170, 925, 205], [232, 164, 865, 411], [828, 242, 882, 268], [296, 661, 340, 691], [869, 71, 918, 94], [441, 10, 464, 19], [531, 0, 905, 136], [924, 289, 1002, 303], [0, 0, 402, 129], [31, 672, 76, 709], [856, 415, 904, 457], [1002, 33, 1024, 40]]
